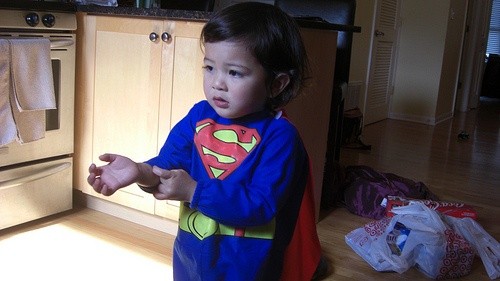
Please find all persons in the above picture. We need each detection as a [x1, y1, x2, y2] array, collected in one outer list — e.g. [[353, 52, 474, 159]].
[[87, 2, 323, 281]]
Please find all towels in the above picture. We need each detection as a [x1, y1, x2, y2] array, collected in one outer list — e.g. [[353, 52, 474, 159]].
[[0, 39, 18, 148], [9, 38, 57, 145]]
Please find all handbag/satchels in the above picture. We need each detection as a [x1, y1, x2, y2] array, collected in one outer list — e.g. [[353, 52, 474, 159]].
[[344, 195, 500, 281]]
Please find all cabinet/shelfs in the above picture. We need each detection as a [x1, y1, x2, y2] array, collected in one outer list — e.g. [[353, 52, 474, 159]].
[[75, 15, 338, 238]]
